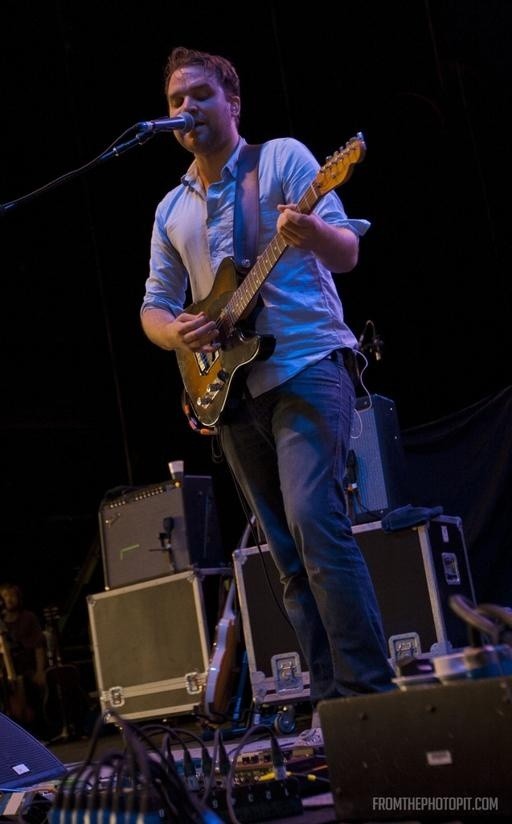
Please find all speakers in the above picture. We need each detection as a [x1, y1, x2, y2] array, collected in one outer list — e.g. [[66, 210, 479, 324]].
[[98, 482, 192, 589], [319, 676, 512, 821]]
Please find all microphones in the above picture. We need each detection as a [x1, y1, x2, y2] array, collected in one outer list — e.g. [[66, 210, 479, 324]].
[[139, 113, 197, 134]]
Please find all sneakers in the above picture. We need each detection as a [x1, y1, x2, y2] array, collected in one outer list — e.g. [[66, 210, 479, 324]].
[[294, 727, 323, 747]]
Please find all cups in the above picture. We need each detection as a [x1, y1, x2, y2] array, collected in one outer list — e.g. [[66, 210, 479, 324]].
[[167, 460, 184, 489]]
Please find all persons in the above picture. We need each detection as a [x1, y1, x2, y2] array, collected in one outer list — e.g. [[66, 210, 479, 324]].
[[139, 48, 398, 767], [2, 581, 48, 738]]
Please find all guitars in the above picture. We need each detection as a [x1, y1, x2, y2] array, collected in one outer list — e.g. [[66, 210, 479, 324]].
[[202, 514, 256, 716], [175, 132, 368, 428]]
